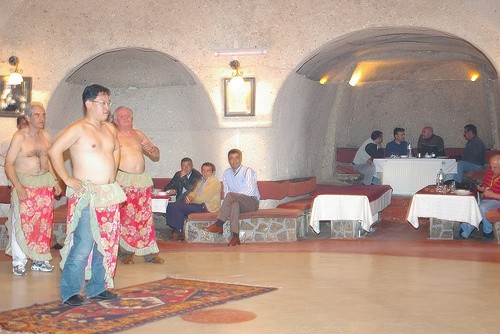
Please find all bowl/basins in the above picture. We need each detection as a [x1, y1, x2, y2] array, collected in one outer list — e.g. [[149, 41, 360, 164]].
[[159, 192, 166, 196]]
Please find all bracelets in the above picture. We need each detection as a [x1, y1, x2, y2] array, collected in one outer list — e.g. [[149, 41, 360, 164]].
[[55, 179, 59, 182], [150, 147, 157, 153]]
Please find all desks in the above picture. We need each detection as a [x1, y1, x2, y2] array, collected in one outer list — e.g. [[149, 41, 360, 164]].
[[151, 189, 176, 237], [309, 185, 393, 238], [407, 185, 482, 240]]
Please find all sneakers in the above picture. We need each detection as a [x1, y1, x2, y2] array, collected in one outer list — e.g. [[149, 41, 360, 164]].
[[90, 290, 118, 300], [64, 294, 90, 306], [149, 256, 163, 264], [13, 265, 26, 275], [31, 262, 55, 272], [122, 254, 134, 264]]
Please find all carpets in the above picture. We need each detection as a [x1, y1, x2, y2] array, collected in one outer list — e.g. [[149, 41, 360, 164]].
[[0, 274, 279, 334]]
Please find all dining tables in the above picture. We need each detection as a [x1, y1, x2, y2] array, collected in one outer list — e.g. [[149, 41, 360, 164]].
[[373, 156, 457, 194]]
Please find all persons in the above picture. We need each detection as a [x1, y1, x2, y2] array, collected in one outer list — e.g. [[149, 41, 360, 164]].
[[385, 128, 415, 157], [444, 124, 486, 189], [202, 148, 259, 246], [48, 84, 127, 306], [417, 126, 444, 158], [164, 157, 221, 241], [113, 106, 164, 264], [459, 153, 500, 239], [8, 116, 63, 249], [352, 131, 386, 185], [5, 102, 62, 275]]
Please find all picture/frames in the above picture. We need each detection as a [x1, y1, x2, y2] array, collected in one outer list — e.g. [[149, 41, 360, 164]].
[[0, 75, 32, 117], [224, 77, 255, 116]]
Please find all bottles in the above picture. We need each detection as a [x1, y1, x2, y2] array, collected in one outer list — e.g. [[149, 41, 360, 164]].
[[407, 140, 413, 159], [436, 169, 444, 189]]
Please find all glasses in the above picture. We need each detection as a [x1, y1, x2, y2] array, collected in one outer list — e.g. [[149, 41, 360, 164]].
[[90, 100, 111, 106]]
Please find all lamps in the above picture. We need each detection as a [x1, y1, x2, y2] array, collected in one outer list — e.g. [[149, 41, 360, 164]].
[[8, 56, 24, 85], [214, 47, 268, 56], [229, 60, 245, 91]]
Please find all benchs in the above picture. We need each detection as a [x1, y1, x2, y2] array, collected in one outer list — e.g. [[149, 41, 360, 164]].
[[276, 197, 314, 239], [184, 209, 304, 243], [0, 204, 66, 247], [337, 166, 360, 186]]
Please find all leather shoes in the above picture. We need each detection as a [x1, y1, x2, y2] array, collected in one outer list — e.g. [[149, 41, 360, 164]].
[[226, 237, 240, 245], [170, 229, 185, 241], [202, 224, 223, 234]]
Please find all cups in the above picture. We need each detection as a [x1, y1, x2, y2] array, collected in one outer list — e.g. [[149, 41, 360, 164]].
[[449, 181, 455, 193], [442, 185, 447, 194]]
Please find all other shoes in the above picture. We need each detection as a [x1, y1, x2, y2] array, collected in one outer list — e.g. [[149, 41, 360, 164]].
[[482, 231, 494, 239], [54, 243, 63, 249]]
